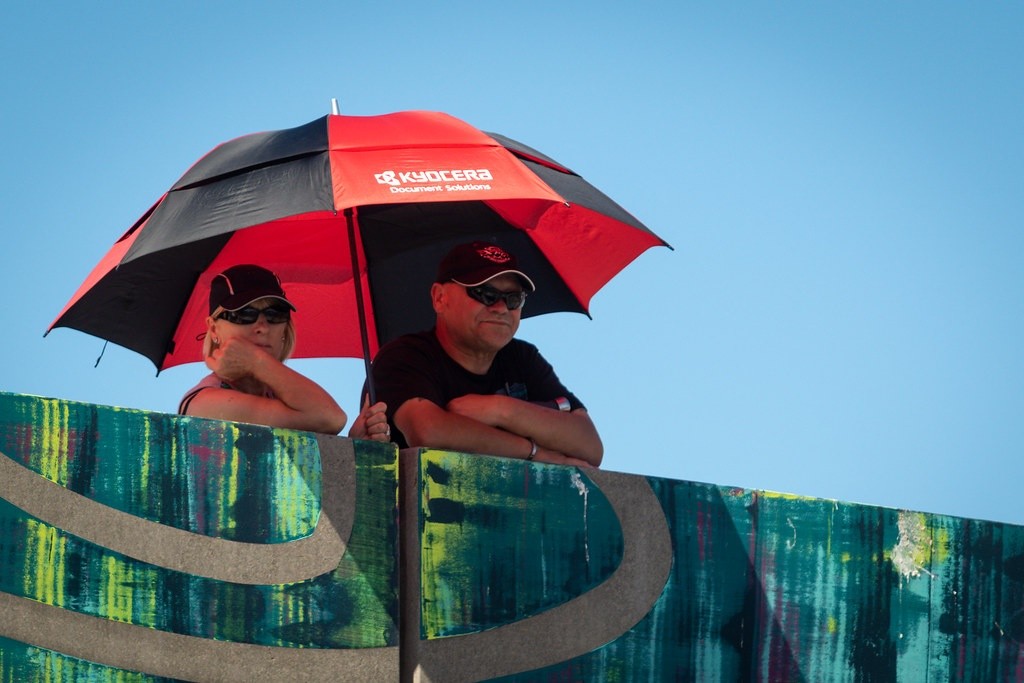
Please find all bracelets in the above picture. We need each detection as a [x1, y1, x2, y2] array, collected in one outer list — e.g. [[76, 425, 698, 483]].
[[526, 437, 537, 460]]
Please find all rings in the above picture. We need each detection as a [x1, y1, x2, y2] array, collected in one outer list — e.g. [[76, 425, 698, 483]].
[[386, 424, 390, 435]]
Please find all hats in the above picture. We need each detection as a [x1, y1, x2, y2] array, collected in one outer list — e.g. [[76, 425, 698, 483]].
[[437, 242, 536, 295], [209, 265, 297, 316]]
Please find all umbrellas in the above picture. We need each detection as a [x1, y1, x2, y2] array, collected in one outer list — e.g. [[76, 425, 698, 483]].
[[44, 98, 675, 407]]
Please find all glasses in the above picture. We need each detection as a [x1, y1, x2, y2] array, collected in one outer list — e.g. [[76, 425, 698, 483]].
[[217, 302, 290, 324], [466, 284, 528, 311]]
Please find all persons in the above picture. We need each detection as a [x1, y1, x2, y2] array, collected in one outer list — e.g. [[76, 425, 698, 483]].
[[179, 264, 390, 443], [360, 240, 604, 470]]
